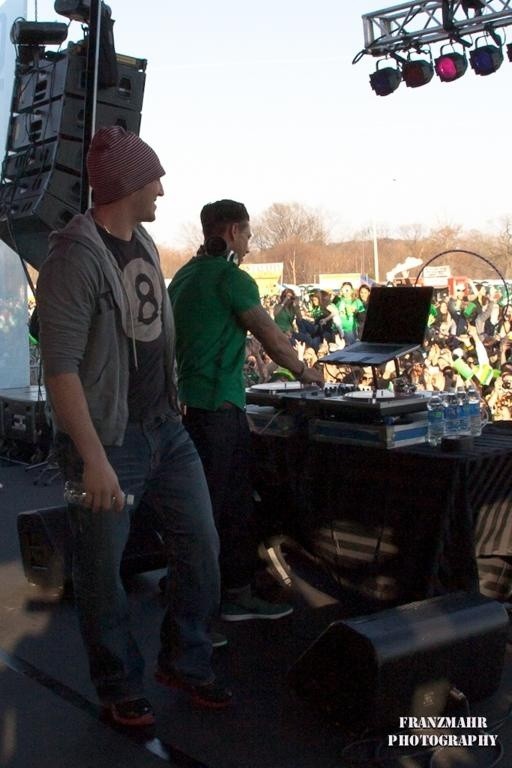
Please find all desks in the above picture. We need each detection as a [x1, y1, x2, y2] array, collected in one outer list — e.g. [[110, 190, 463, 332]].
[[236, 432, 512, 605]]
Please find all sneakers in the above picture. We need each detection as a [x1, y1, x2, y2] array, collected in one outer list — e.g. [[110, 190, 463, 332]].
[[221, 585, 295, 621], [97, 684, 157, 729], [152, 655, 237, 708], [205, 629, 229, 646]]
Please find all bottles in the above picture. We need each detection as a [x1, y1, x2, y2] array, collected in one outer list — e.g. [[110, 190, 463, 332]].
[[63, 480, 135, 509], [466, 384, 482, 437], [426, 391, 444, 447], [455, 386, 471, 436], [442, 387, 461, 440]]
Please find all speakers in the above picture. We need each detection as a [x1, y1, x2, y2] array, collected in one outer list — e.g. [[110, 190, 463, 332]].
[[1, 43, 147, 272]]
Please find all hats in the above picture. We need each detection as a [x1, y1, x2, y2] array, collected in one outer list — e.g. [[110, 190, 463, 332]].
[[85, 125, 166, 206]]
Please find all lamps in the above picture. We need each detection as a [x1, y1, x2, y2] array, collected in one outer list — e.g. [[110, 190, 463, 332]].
[[469, 21, 507, 76], [402, 43, 436, 86], [434, 34, 468, 82], [369, 46, 401, 96]]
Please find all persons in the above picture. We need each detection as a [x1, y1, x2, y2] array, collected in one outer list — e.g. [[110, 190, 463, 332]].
[[39, 126, 233, 725], [166, 198, 324, 650], [243, 280, 511, 422]]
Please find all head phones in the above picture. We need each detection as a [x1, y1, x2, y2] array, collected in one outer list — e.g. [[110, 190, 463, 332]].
[[196, 236, 239, 266]]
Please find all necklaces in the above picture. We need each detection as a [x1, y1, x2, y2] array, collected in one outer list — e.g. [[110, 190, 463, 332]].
[[93, 213, 110, 236]]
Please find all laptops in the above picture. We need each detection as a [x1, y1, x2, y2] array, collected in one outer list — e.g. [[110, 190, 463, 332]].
[[318, 285, 435, 366]]
